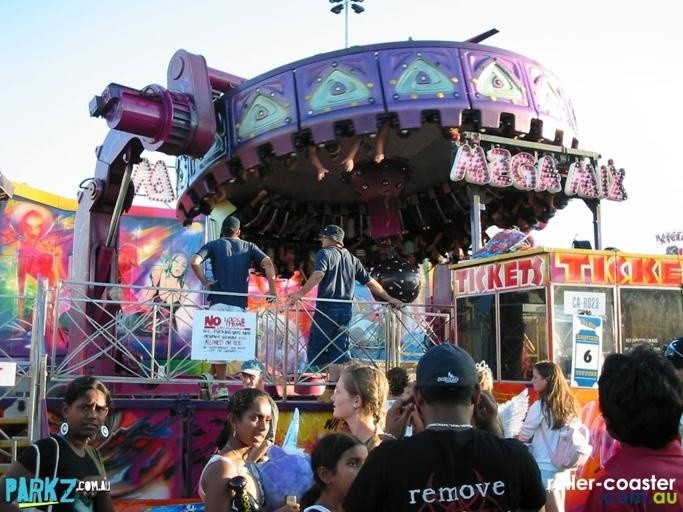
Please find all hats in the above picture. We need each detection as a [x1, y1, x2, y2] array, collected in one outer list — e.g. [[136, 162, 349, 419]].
[[415, 343, 478, 388], [236, 360, 266, 375], [318, 225, 345, 246], [222, 216, 240, 233]]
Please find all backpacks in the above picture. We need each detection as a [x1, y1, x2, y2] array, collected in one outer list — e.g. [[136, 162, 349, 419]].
[[540, 418, 593, 471]]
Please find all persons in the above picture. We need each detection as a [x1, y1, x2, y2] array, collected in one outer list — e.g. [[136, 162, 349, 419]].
[[203, 121, 576, 272], [127, 254, 189, 336], [191, 215, 277, 387], [0, 376, 116, 512], [285, 224, 406, 370], [197, 342, 681, 511]]
